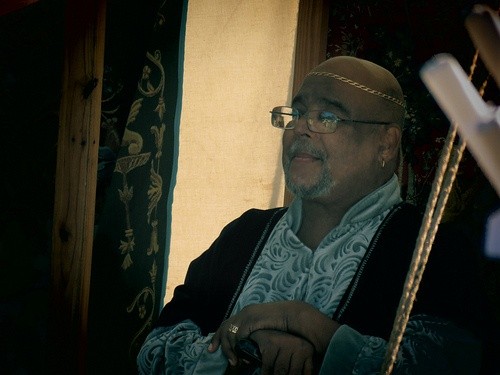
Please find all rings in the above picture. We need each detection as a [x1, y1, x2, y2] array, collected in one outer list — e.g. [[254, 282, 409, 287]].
[[228, 324, 239, 334]]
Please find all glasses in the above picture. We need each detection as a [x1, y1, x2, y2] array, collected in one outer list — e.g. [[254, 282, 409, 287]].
[[269, 105, 391, 133]]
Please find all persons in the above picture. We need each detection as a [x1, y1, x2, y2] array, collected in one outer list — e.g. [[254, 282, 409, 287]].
[[130, 54, 485, 375]]
